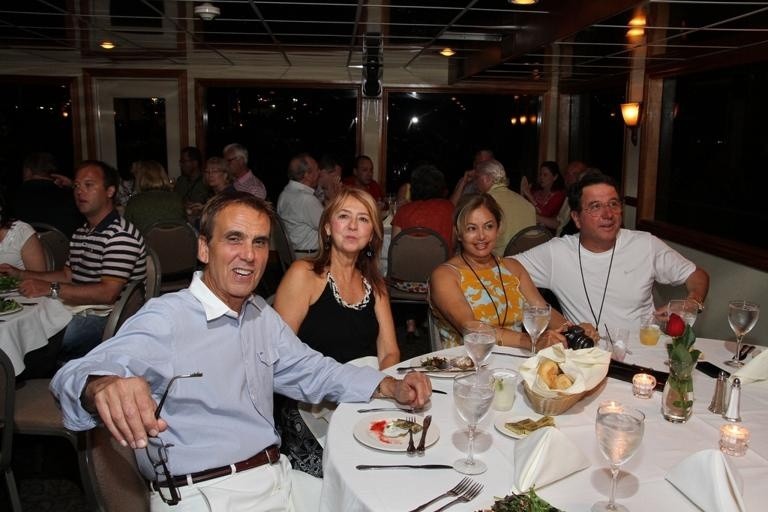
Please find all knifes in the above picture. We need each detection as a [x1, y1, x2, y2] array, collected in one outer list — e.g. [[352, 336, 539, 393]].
[[740, 346, 756, 361], [732, 344, 748, 360], [416, 416, 437, 463], [355, 463, 453, 471], [432, 390, 446, 395]]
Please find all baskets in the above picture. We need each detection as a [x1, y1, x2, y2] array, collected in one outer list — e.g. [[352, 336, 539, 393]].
[[520, 380, 587, 417]]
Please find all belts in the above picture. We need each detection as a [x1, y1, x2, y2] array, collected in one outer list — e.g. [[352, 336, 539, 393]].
[[146, 443, 281, 493], [293, 248, 317, 254]]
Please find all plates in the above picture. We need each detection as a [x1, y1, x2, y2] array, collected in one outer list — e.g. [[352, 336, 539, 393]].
[[353, 407, 439, 454], [495, 412, 546, 440], [411, 356, 485, 379], [0, 304, 22, 316]]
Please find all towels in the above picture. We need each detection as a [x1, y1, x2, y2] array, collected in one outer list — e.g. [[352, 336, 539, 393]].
[[664, 447, 746, 512], [727, 348, 767, 389], [512, 425, 593, 494]]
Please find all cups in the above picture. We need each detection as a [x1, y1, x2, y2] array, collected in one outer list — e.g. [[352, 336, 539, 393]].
[[490, 367, 519, 411], [453, 374, 496, 475], [462, 324, 496, 383], [669, 298, 696, 329], [522, 303, 551, 361], [605, 327, 628, 362], [724, 300, 761, 369], [596, 402, 646, 512], [640, 314, 659, 346]]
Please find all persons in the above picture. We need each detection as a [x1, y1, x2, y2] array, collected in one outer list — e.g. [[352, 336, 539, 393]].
[[449, 147, 494, 207], [314, 157, 342, 204], [49, 189, 432, 512], [277, 154, 343, 260], [536, 160, 588, 238], [390, 165, 457, 257], [168, 147, 206, 205], [476, 158, 536, 257], [222, 143, 267, 201], [0, 191, 47, 278], [428, 193, 601, 353], [342, 155, 386, 202], [186, 157, 238, 230], [117, 160, 187, 235], [0, 160, 147, 366], [505, 171, 711, 338], [275, 189, 400, 373], [520, 161, 567, 236]]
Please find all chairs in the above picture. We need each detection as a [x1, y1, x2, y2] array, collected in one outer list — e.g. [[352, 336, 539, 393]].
[[386, 226, 449, 300], [14, 279, 145, 434], [503, 224, 556, 257], [144, 241, 162, 301], [144, 219, 200, 289], [0, 348, 22, 510], [274, 209, 297, 271], [78, 424, 149, 512]]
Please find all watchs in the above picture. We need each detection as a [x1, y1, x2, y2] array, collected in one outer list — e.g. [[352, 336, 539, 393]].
[[50, 282, 60, 299], [686, 296, 703, 313]]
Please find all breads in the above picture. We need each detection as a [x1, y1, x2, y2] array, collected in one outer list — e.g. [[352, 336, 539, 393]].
[[554, 373, 573, 389], [538, 360, 558, 389]]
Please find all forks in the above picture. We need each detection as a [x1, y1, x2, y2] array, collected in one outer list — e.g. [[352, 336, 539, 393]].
[[397, 360, 429, 372], [436, 481, 485, 512], [405, 416, 415, 454], [409, 474, 474, 512]]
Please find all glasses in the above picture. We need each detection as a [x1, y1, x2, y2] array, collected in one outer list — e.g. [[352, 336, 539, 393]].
[[144, 371, 205, 507], [582, 199, 622, 218]]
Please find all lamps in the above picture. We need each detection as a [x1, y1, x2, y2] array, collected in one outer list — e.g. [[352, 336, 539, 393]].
[[619, 97, 647, 147]]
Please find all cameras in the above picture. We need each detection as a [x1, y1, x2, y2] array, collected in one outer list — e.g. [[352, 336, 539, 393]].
[[559, 323, 595, 350]]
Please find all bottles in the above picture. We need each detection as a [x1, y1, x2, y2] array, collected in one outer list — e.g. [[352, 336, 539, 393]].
[[709, 371, 725, 414], [722, 379, 741, 424]]
